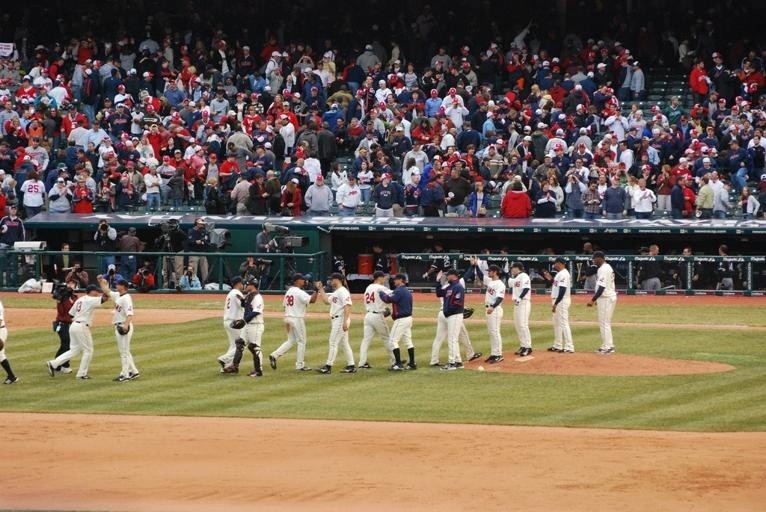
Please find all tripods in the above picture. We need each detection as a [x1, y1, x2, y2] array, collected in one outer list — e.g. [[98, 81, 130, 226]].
[[152, 240, 181, 289], [199, 254, 235, 293], [263, 254, 304, 293]]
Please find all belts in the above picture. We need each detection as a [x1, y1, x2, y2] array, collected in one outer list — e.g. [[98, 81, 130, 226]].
[[331, 315, 340, 319]]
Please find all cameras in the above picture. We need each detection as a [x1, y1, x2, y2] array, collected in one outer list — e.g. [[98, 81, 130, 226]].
[[186, 271, 192, 276], [156, 224, 177, 240], [256, 258, 273, 265], [143, 268, 149, 276], [75, 268, 83, 273], [270, 225, 309, 253], [109, 269, 114, 275], [53, 283, 73, 301], [101, 224, 107, 231], [204, 222, 231, 248]]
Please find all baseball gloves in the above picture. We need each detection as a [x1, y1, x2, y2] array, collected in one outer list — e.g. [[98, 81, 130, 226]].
[[383, 307, 392, 318], [463, 307, 475, 320]]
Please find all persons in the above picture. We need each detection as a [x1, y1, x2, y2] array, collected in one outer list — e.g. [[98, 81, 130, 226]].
[[479, 248, 490, 255], [1, 1, 766, 221], [52, 279, 78, 374], [359, 272, 407, 368], [179, 267, 202, 291], [255, 221, 276, 281], [734, 253, 752, 290], [422, 241, 469, 285], [188, 219, 210, 286], [715, 244, 735, 290], [579, 243, 599, 290], [65, 263, 89, 289], [378, 273, 417, 371], [485, 265, 505, 364], [46, 279, 109, 379], [314, 273, 357, 373], [373, 245, 391, 278], [507, 262, 534, 357], [436, 270, 465, 370], [101, 264, 126, 289], [97, 275, 140, 383], [544, 258, 574, 353], [0, 204, 25, 256], [538, 246, 560, 288], [673, 248, 701, 289], [498, 245, 520, 290], [134, 262, 155, 292], [477, 249, 498, 280], [153, 219, 187, 292], [635, 247, 661, 291], [586, 251, 618, 354], [222, 275, 264, 377], [118, 227, 145, 281], [0, 298, 19, 384], [429, 266, 482, 366], [217, 277, 246, 373], [94, 218, 117, 274], [235, 254, 259, 287], [371, 241, 408, 287], [269, 274, 319, 371]]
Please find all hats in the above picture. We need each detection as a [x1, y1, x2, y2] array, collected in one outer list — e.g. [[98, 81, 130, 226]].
[[738, 114, 747, 119], [731, 105, 739, 112], [266, 115, 274, 120], [151, 124, 157, 130], [127, 161, 135, 170], [357, 91, 363, 98], [256, 136, 264, 144], [163, 156, 172, 161], [142, 45, 148, 50], [596, 64, 606, 68], [125, 141, 133, 147], [485, 265, 501, 272], [536, 109, 545, 114], [587, 38, 596, 44], [264, 142, 271, 149], [121, 134, 128, 140], [603, 135, 613, 140], [69, 99, 82, 109], [486, 112, 492, 119], [272, 51, 281, 57], [486, 51, 493, 57], [0, 96, 8, 104], [649, 106, 660, 112], [491, 44, 497, 49], [264, 86, 272, 92], [412, 140, 420, 145], [496, 139, 503, 144], [117, 322, 129, 334], [327, 272, 343, 280], [611, 176, 622, 182], [57, 177, 64, 184], [235, 93, 244, 99], [587, 72, 594, 78], [283, 89, 289, 96], [166, 218, 181, 226], [701, 146, 709, 154], [131, 136, 138, 142], [108, 264, 116, 269], [246, 278, 258, 286], [23, 75, 30, 80], [230, 276, 242, 286], [396, 82, 402, 87], [291, 179, 300, 186], [316, 176, 325, 181], [365, 44, 375, 50], [149, 163, 156, 170], [147, 105, 154, 112], [331, 104, 338, 108], [710, 91, 719, 96], [612, 134, 618, 140], [730, 126, 740, 132], [381, 171, 394, 180], [116, 103, 124, 108], [559, 114, 566, 121], [629, 127, 638, 132], [102, 163, 109, 167], [462, 46, 469, 52], [39, 85, 47, 90], [600, 168, 606, 175], [101, 173, 110, 179], [118, 85, 125, 91], [741, 101, 752, 107], [712, 52, 723, 59], [736, 96, 743, 103], [684, 174, 694, 182], [614, 107, 624, 111], [373, 271, 388, 280], [392, 273, 407, 281], [679, 157, 690, 164], [589, 53, 596, 58], [192, 78, 201, 83], [490, 181, 496, 187], [543, 61, 550, 66], [538, 123, 544, 129], [188, 137, 195, 143], [593, 45, 599, 51], [450, 88, 456, 96], [691, 139, 698, 144], [379, 101, 386, 109], [702, 158, 711, 164], [553, 66, 560, 71], [555, 144, 563, 150], [523, 136, 532, 141], [172, 111, 181, 119], [614, 42, 622, 47], [120, 173, 129, 182], [56, 74, 64, 80], [109, 151, 114, 159], [187, 66, 196, 75], [453, 151, 460, 158], [707, 127, 715, 132], [57, 164, 66, 171], [304, 68, 312, 73], [711, 149, 718, 157], [8, 178, 16, 188], [552, 257, 566, 264], [0, 169, 4, 175], [227, 110, 236, 117], [575, 85, 582, 90], [431, 89, 437, 97], [524, 126, 531, 130], [348, 172, 356, 179], [202, 110, 209, 118], [370, 108, 378, 114], [8, 205, 18, 209], [174, 150, 183, 155], [250, 93, 258, 100], [23, 155, 31, 160], [509, 263, 523, 268], [606, 87, 615, 94], [553, 58, 559, 63], [195, 218, 207, 225], [443, 270, 460, 277], [556, 129, 563, 135], [188, 102, 195, 107], [209, 153, 216, 159], [633, 61, 639, 67], [86, 285, 101, 290], [282, 102, 289, 106], [452, 99, 460, 104], [391, 75, 396, 83], [719, 98, 727, 104], [488, 100, 494, 106], [545, 154, 552, 159], [577, 143, 587, 148], [279, 115, 287, 120], [580, 128, 587, 133], [35, 45, 44, 50], [489, 145, 495, 149], [311, 87, 319, 92], [463, 62, 470, 69], [635, 110, 645, 116], [93, 59, 101, 66], [294, 93, 300, 98], [40, 69, 48, 73], [130, 68, 137, 75], [30, 160, 38, 167], [690, 129, 699, 135], [411, 168, 422, 177], [591, 252, 605, 259], [168, 80, 176, 88], [685, 149, 695, 154], [104, 98, 111, 102], [619, 162, 625, 168], [598, 40, 605, 46], [643, 136, 650, 142], [434, 60, 443, 66], [441, 161, 450, 168], [433, 155, 442, 161], [143, 72, 154, 77], [577, 104, 583, 110], [728, 140, 740, 145], [641, 164, 654, 171], [144, 96, 153, 104], [394, 60, 401, 64], [292, 272, 312, 281], [85, 69, 92, 75]]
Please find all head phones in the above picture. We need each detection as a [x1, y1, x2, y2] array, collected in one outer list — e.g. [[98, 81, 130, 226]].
[[261, 221, 271, 229]]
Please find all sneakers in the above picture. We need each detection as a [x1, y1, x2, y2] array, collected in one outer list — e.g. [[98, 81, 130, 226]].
[[269, 355, 278, 370], [484, 354, 504, 365], [297, 366, 311, 371], [77, 375, 93, 380], [315, 364, 333, 374], [47, 361, 55, 378], [340, 364, 357, 374], [387, 359, 417, 371], [515, 347, 532, 357], [548, 347, 574, 354], [248, 371, 262, 378], [4, 375, 18, 385], [595, 346, 616, 354], [128, 373, 140, 381], [430, 361, 464, 372], [469, 353, 482, 362], [217, 359, 239, 374], [114, 375, 131, 383], [359, 363, 374, 369], [61, 367, 72, 375]]
